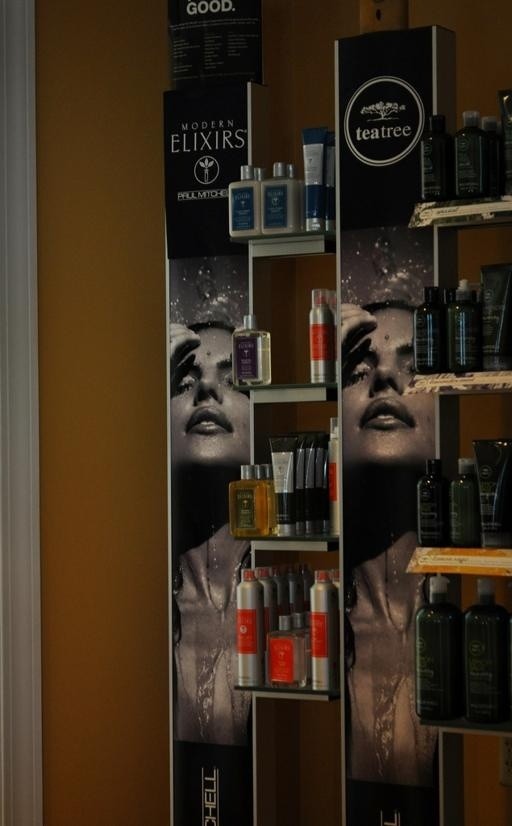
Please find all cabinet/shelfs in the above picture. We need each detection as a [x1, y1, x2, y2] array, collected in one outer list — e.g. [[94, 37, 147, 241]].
[[227, 236, 338, 703], [408, 196, 511, 737]]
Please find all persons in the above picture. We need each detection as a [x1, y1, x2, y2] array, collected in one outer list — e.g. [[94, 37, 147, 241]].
[[169, 318, 254, 744], [341, 296, 436, 785]]
